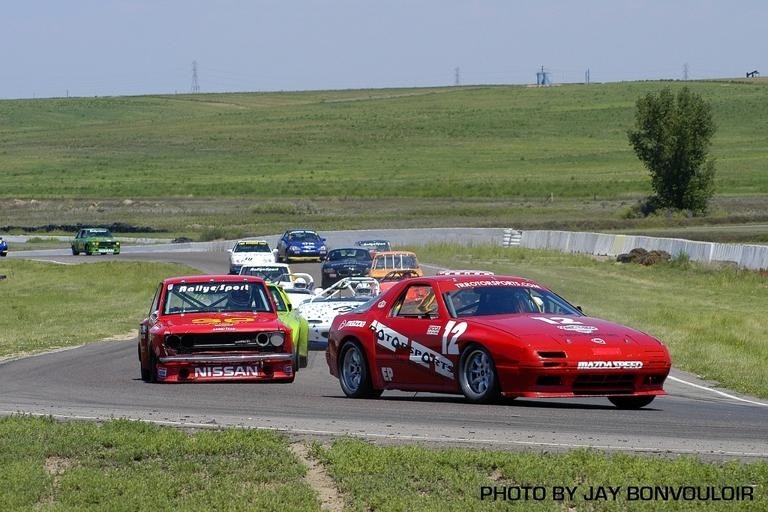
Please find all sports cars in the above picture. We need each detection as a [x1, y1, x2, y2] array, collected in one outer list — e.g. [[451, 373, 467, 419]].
[[0, 238, 8, 256], [71, 227, 121, 257]]
